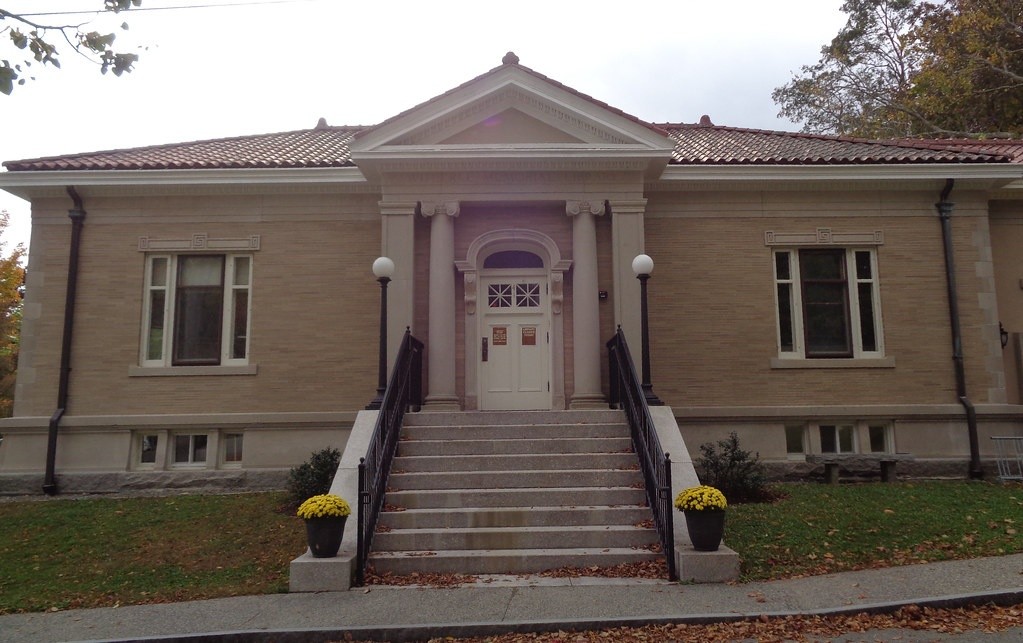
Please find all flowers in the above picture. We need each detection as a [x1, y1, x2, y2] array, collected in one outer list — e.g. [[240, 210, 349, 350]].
[[673, 485, 728, 511], [296, 494, 352, 521]]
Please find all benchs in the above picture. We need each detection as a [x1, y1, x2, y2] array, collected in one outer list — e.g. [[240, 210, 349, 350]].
[[804, 455, 904, 485]]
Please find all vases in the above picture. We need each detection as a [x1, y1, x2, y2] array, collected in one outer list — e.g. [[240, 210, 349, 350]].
[[305, 516, 348, 558], [684, 510, 726, 551]]
